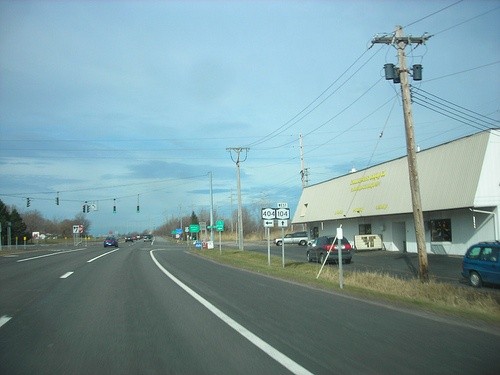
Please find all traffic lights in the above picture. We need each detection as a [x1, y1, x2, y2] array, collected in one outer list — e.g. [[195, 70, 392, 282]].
[[56, 197, 59, 206], [27, 198, 30, 207], [113, 205, 116, 213], [87, 205, 90, 213], [82, 205, 86, 213], [136, 205, 140, 213]]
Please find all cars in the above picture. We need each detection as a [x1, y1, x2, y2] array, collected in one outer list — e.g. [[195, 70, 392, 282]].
[[132, 236, 137, 240], [125, 236, 133, 243], [103, 237, 119, 248], [136, 234, 153, 241]]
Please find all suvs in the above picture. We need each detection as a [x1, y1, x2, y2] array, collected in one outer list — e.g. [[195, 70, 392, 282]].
[[273, 231, 308, 246], [304, 235, 352, 264], [460, 240, 500, 288]]
